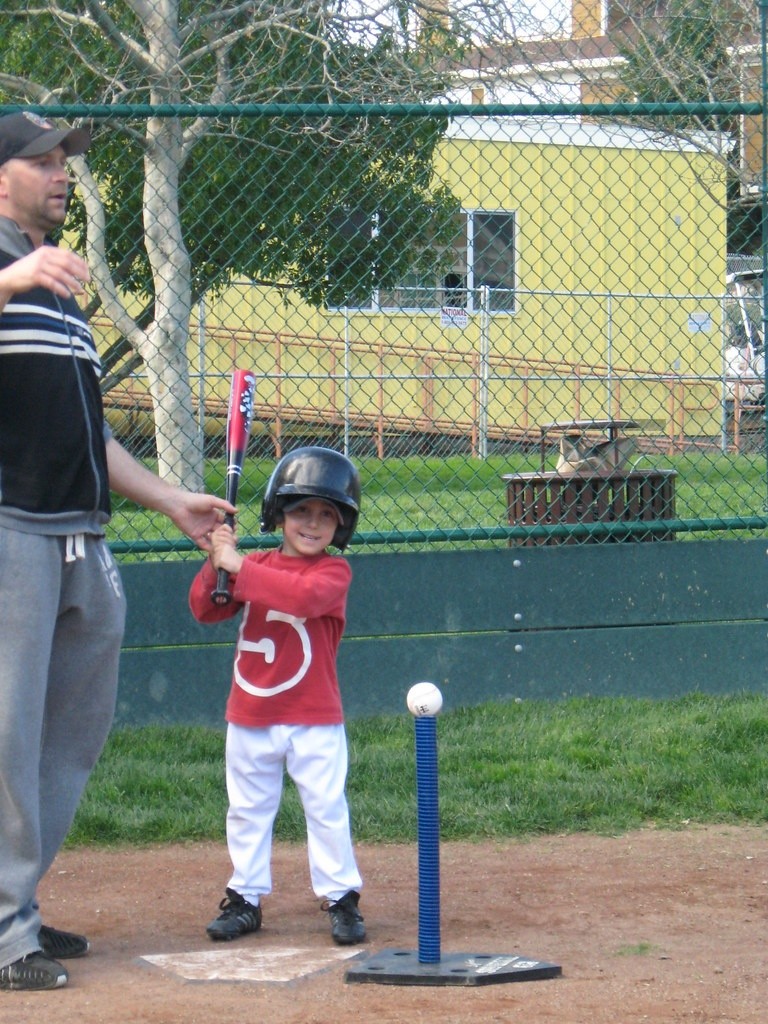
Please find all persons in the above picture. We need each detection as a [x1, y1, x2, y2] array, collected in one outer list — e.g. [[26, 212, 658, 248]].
[[0, 112, 238, 990], [189, 447, 366, 946]]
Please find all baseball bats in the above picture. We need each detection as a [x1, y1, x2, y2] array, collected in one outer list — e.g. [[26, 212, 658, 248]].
[[210, 363, 257, 603]]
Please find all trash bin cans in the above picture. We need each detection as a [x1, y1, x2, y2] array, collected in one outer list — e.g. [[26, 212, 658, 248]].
[[500, 418, 679, 548]]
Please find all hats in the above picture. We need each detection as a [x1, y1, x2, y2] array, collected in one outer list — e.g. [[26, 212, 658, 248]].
[[0, 111, 92, 168]]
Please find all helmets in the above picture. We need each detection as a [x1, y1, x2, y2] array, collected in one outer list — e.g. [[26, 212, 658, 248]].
[[258, 445, 360, 553]]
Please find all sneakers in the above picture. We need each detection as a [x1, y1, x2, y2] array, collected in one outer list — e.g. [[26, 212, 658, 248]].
[[0, 953, 68, 992], [35, 925, 90, 959], [207, 888, 262, 940], [321, 889, 368, 945]]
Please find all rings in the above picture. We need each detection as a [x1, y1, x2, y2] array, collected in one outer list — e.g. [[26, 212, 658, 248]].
[[206, 532, 211, 540]]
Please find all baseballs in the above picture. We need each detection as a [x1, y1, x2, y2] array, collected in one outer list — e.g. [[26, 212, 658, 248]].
[[404, 681, 448, 718]]
